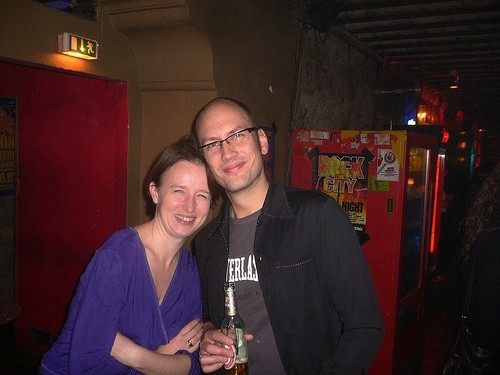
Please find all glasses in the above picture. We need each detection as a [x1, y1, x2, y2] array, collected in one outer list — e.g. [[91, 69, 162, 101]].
[[198, 127, 258, 156]]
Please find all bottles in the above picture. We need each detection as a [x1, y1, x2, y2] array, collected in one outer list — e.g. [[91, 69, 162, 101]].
[[220, 282, 250, 375]]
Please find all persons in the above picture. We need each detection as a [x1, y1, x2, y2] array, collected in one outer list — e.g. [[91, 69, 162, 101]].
[[440, 178, 476, 250], [440, 167, 500, 375], [191, 97, 385, 375], [38, 136, 220, 374]]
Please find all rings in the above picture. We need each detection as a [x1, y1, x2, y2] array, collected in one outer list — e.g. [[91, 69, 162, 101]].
[[186, 340, 193, 348], [199, 352, 205, 360]]
